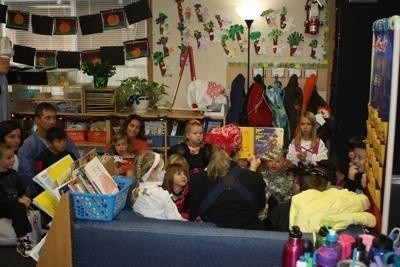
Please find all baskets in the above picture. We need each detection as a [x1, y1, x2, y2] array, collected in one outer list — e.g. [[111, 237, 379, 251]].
[[64, 130, 87, 142], [70, 177, 133, 221], [88, 130, 107, 143]]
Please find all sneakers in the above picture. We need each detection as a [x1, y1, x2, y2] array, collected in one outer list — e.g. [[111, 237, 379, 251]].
[[16, 236, 33, 259]]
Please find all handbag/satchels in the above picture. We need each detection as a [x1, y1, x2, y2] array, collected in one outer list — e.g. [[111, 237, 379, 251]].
[[246, 81, 275, 127], [266, 80, 289, 128]]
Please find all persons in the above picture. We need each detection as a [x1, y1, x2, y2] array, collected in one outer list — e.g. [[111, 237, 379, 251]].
[[131, 149, 188, 221], [31, 126, 77, 230], [17, 102, 81, 178], [0, 120, 36, 247], [183, 110, 377, 233], [168, 153, 190, 173], [0, 36, 14, 122], [163, 163, 190, 219], [120, 113, 148, 152], [108, 131, 136, 177], [0, 143, 34, 257]]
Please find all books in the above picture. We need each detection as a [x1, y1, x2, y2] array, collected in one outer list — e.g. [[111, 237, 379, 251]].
[[32, 154, 122, 219]]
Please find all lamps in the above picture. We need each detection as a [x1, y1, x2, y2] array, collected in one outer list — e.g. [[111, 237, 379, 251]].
[[235, 0, 264, 92]]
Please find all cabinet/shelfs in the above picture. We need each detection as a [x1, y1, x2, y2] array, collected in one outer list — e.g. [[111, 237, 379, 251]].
[[8, 111, 204, 166]]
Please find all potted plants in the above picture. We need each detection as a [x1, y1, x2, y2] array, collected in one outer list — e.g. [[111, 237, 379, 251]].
[[82, 60, 117, 87], [119, 76, 169, 114]]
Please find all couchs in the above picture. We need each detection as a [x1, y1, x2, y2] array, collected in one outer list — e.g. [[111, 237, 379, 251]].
[[72, 212, 321, 267]]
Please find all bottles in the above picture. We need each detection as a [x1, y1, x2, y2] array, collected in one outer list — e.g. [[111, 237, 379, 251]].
[[282, 224, 400, 267]]
